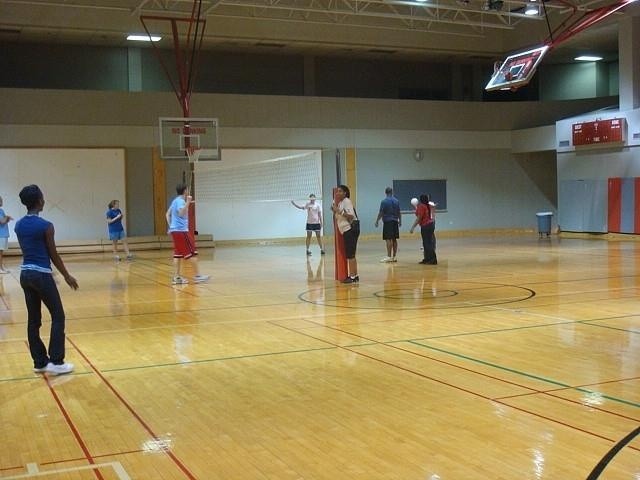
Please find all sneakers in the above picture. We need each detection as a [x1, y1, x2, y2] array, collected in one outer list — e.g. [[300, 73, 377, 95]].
[[380, 257, 397, 262], [343, 274, 359, 283], [172, 276, 189, 285], [193, 275, 208, 283], [321, 249, 325, 254], [34, 361, 74, 373], [307, 249, 312, 255]]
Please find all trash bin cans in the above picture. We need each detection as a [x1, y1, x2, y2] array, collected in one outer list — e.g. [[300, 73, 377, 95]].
[[536, 212, 553, 236]]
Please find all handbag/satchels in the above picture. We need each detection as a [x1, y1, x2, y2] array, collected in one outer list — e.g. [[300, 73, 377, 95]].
[[351, 220, 360, 236]]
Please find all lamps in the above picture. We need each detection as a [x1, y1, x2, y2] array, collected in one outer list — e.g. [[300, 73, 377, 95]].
[[524, 0, 539, 15]]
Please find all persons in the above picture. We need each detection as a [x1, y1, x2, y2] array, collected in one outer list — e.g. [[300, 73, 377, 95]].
[[14, 183, 81, 376], [290, 193, 329, 255], [0, 191, 14, 274], [331, 184, 360, 284], [164, 182, 213, 286], [413, 192, 441, 251], [410, 192, 438, 265], [374, 186, 403, 262], [104, 198, 139, 261]]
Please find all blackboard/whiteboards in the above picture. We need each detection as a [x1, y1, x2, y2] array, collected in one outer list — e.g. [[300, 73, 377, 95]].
[[393, 180, 446, 213]]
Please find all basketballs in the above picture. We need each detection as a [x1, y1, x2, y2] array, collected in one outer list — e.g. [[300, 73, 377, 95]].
[[411, 198, 418, 206]]
